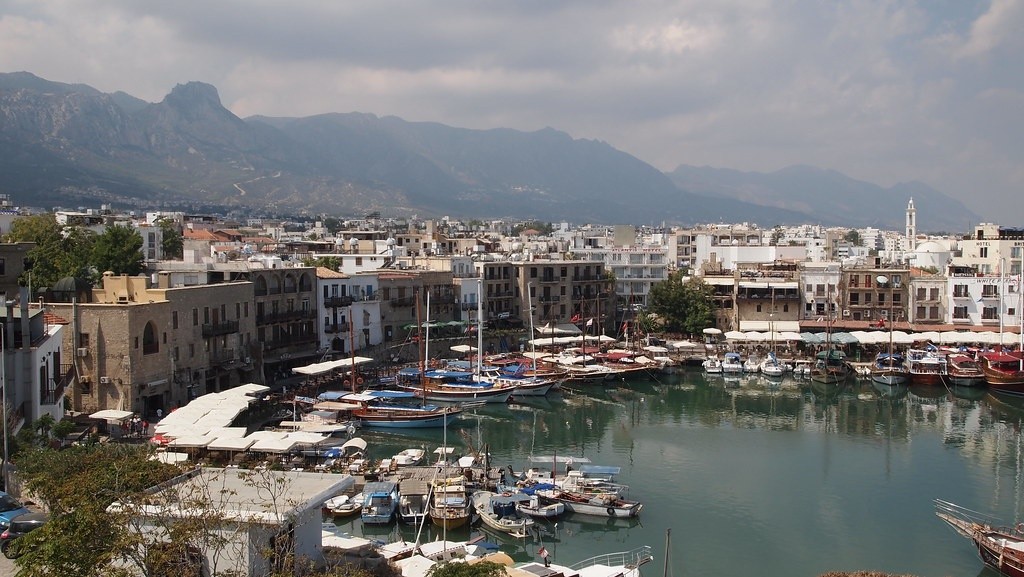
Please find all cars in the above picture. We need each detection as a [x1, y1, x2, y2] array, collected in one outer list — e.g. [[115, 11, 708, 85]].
[[0, 491, 31, 536], [465, 320, 490, 334], [1, 514, 49, 559]]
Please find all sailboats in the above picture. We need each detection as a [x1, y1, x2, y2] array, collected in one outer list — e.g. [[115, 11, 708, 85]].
[[396, 280, 667, 403], [317, 292, 464, 428], [811, 284, 853, 384], [373, 406, 480, 577], [871, 320, 911, 385], [976, 258, 1024, 391]]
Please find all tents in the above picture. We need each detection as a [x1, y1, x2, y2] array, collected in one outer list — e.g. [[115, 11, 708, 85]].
[[725, 330, 1024, 351], [703, 328, 722, 335], [154, 383, 328, 462], [88, 409, 134, 436], [292, 356, 374, 379]]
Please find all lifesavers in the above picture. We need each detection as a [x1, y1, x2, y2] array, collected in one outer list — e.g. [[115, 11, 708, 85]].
[[356, 378, 363, 384], [344, 380, 350, 386], [607, 506, 615, 516]]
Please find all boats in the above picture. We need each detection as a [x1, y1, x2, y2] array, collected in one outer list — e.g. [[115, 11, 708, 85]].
[[323, 495, 349, 514], [497, 484, 564, 516], [947, 354, 986, 385], [429, 478, 471, 530], [514, 545, 654, 576], [398, 495, 429, 526], [906, 349, 948, 385], [471, 490, 534, 532], [540, 465, 643, 517], [513, 456, 591, 486], [721, 353, 743, 372], [794, 365, 811, 375], [761, 354, 785, 376], [332, 492, 364, 516], [392, 449, 425, 465], [701, 355, 722, 373], [932, 498, 1024, 577], [744, 358, 760, 372]]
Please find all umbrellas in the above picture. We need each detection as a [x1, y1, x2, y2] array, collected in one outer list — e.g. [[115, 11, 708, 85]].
[[403, 320, 472, 333]]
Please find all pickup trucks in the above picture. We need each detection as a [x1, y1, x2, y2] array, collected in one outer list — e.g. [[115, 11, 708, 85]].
[[409, 331, 437, 340]]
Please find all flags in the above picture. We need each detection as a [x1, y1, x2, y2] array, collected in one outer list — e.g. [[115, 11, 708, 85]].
[[570, 314, 580, 323], [543, 322, 549, 330], [587, 318, 592, 325]]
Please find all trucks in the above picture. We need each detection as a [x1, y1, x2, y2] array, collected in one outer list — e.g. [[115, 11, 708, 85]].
[[361, 482, 401, 523]]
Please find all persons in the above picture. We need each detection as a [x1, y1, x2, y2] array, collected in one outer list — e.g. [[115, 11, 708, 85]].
[[706, 334, 715, 344], [119, 415, 149, 438], [156, 408, 163, 418]]
